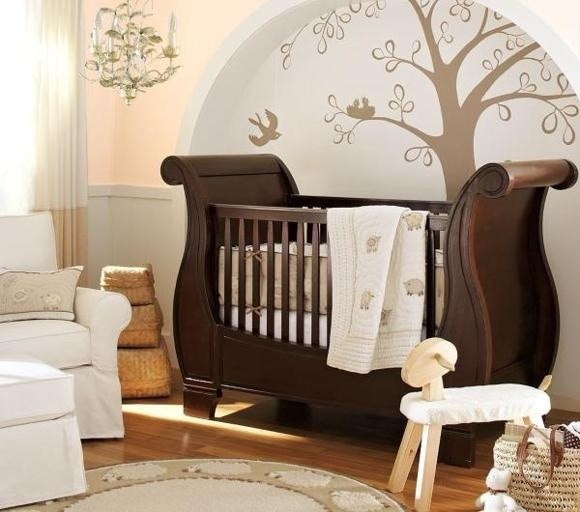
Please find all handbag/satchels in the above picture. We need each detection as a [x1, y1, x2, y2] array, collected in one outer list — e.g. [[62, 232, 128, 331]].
[[492, 420, 580, 512]]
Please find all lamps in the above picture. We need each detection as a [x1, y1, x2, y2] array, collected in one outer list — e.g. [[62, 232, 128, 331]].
[[80, 0, 180, 106]]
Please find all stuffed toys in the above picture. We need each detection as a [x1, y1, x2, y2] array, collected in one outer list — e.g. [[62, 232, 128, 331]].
[[475, 467, 518, 512]]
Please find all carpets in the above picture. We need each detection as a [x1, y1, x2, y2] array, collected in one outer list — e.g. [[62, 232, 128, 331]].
[[0, 457, 414, 512]]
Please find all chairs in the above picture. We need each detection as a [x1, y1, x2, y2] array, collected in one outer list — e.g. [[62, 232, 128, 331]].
[[0, 208, 133, 441]]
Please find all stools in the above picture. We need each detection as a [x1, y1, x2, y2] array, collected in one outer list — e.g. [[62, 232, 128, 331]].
[[0, 342, 90, 509], [386, 338, 552, 512]]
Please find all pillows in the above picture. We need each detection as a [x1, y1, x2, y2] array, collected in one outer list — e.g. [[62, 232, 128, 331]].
[[0, 265, 84, 321]]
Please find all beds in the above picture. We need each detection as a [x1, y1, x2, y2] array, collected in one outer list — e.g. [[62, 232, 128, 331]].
[[161, 153, 579, 469]]
